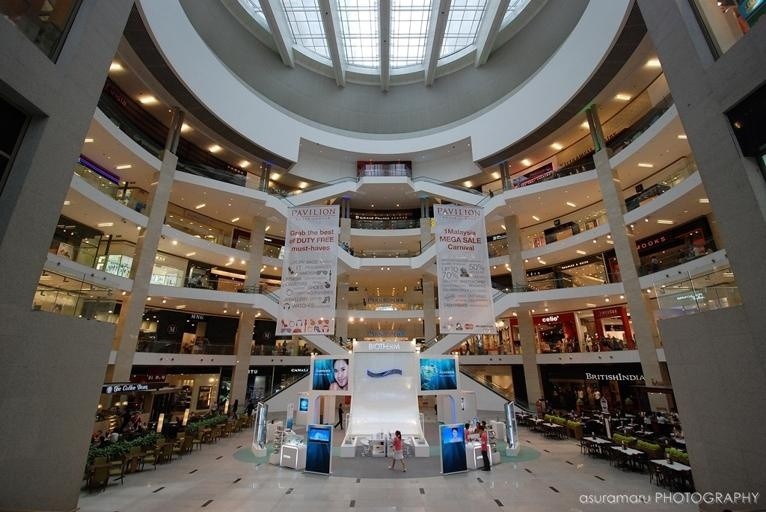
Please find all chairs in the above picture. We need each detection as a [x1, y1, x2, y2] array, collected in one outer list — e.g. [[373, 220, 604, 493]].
[[85, 411, 255, 493]]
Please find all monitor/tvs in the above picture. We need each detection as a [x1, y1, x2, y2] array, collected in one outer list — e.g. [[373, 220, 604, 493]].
[[442, 428, 463, 443], [309, 429, 329, 441]]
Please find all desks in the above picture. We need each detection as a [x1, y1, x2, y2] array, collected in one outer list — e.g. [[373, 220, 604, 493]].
[[515, 409, 696, 492]]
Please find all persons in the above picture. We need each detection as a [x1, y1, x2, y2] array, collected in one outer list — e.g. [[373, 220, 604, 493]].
[[329, 359, 349, 391], [554, 333, 624, 354], [678, 243, 713, 263], [339, 337, 343, 346], [90, 409, 149, 446], [183, 336, 210, 354], [434, 395, 437, 415], [232, 400, 238, 419], [464, 341, 472, 355], [334, 403, 344, 430], [448, 428, 462, 442], [343, 242, 354, 256], [283, 340, 287, 355], [464, 420, 491, 472], [651, 255, 658, 274], [535, 387, 685, 447], [245, 399, 255, 417], [489, 188, 494, 199], [189, 274, 208, 288], [175, 416, 182, 428], [388, 430, 407, 472], [211, 400, 225, 417]]
